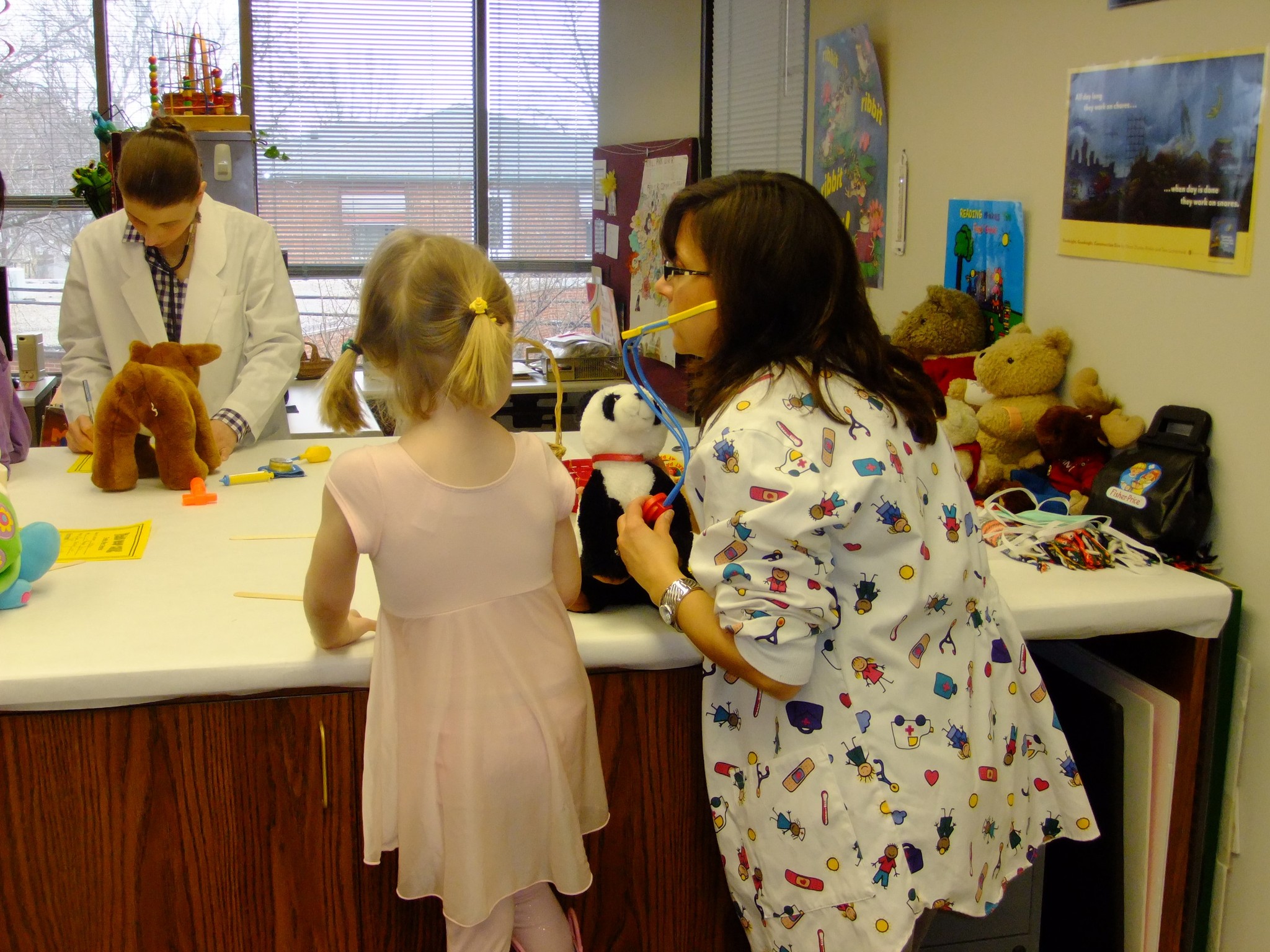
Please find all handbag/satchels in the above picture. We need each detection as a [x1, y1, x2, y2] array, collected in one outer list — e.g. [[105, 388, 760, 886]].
[[1079, 405, 1214, 558]]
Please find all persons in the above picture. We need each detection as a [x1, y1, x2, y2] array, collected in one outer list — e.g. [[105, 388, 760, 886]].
[[612, 168, 1107, 951], [300, 224, 611, 952], [56, 114, 306, 473], [0, 169, 34, 481]]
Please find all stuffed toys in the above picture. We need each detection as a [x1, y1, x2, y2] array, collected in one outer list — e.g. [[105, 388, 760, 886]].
[[937, 319, 1072, 495], [993, 367, 1146, 522], [0, 463, 61, 610], [888, 283, 987, 404], [90, 339, 224, 491], [575, 374, 698, 614]]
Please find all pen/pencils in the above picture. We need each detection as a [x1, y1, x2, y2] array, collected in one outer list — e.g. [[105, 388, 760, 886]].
[[83, 379, 96, 424]]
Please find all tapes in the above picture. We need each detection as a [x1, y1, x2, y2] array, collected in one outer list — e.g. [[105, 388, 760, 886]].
[[269, 458, 294, 472]]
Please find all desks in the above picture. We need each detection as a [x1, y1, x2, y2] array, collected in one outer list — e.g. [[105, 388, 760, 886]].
[[13, 376, 62, 447], [284, 370, 384, 439], [491, 359, 632, 432], [0, 427, 1247, 951]]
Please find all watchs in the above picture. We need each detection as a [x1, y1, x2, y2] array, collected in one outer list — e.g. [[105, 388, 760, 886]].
[[658, 577, 705, 633]]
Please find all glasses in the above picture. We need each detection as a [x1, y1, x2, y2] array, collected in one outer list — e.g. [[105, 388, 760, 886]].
[[663, 259, 712, 282]]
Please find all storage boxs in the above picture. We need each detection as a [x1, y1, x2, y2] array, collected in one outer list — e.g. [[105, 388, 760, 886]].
[[525, 345, 625, 382]]
